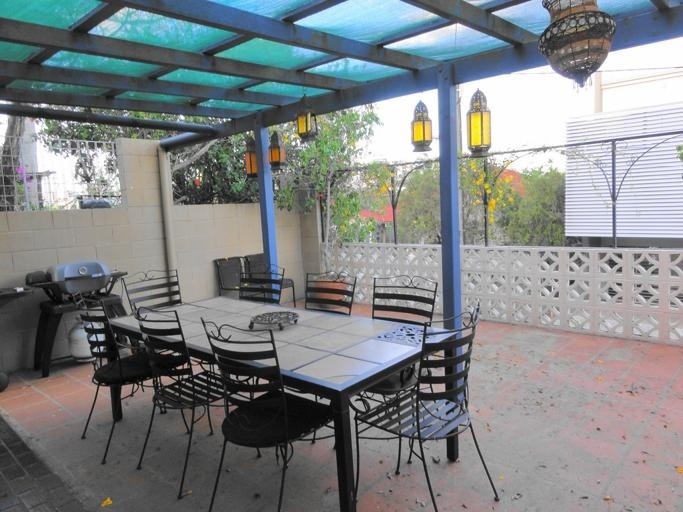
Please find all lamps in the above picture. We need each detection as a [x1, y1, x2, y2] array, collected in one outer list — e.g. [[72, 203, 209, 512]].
[[466, 88, 491, 157], [296, 93, 319, 142], [244, 136, 258, 178], [267, 130, 286, 171], [539, 0, 617, 87], [412, 100, 432, 152]]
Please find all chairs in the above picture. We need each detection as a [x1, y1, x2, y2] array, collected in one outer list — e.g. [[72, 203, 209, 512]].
[[352, 298, 499, 512], [121, 267, 183, 315], [200, 316, 335, 511], [80, 293, 189, 467], [239, 261, 286, 306], [333, 275, 441, 476], [213, 256, 267, 305], [130, 301, 263, 499], [245, 254, 297, 308], [305, 270, 357, 445]]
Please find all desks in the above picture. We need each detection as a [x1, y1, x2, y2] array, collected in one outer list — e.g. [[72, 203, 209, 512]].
[[107, 296, 439, 501]]
